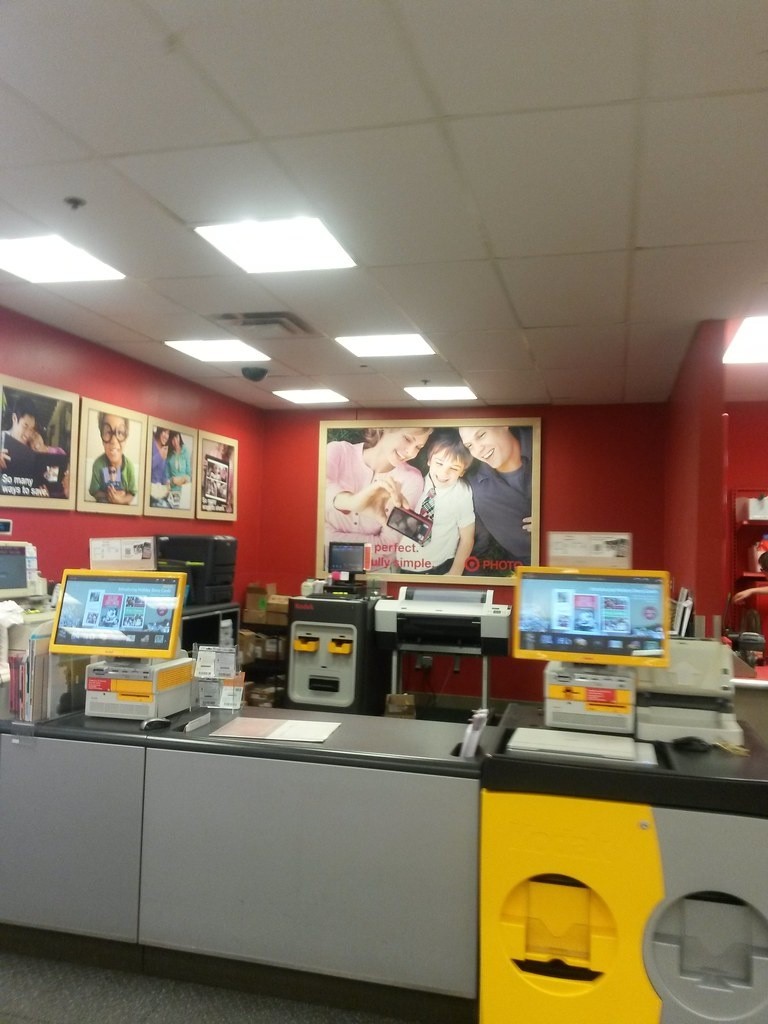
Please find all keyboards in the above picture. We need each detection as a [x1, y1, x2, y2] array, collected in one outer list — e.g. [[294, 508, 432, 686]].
[[306, 593, 357, 600]]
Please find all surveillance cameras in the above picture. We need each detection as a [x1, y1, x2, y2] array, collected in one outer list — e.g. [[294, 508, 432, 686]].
[[242, 367, 268, 382]]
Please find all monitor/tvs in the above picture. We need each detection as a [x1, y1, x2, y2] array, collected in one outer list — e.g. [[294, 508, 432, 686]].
[[49, 568, 187, 660], [328, 541, 366, 586], [512, 565, 671, 668], [0, 540, 38, 598]]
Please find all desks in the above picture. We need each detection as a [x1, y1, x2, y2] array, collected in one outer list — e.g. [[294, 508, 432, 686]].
[[0, 608, 56, 660], [181, 601, 241, 646]]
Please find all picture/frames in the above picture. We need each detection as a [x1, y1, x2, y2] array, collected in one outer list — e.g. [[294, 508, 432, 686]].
[[316, 418, 541, 586], [75, 396, 148, 516], [196, 429, 238, 521], [144, 415, 198, 519], [0, 373, 79, 511], [205, 454, 229, 504]]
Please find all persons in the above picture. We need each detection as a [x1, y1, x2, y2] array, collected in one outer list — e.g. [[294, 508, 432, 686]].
[[393, 429, 475, 578], [89, 411, 138, 505], [26, 427, 71, 500], [56, 593, 170, 646], [162, 430, 192, 509], [522, 590, 661, 649], [106, 466, 121, 491], [731, 551, 768, 606], [459, 425, 534, 568], [149, 426, 174, 509], [0, 395, 70, 499], [325, 426, 435, 573]]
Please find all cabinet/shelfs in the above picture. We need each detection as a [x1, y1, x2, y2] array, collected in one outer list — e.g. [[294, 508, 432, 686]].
[[239, 622, 289, 708], [731, 488, 768, 585]]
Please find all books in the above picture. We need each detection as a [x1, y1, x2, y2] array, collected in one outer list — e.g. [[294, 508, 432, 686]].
[[6, 633, 89, 726]]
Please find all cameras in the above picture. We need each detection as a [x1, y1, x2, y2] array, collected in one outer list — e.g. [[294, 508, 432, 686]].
[[386, 506, 433, 545]]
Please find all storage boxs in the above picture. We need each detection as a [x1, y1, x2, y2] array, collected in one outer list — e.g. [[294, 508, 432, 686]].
[[221, 619, 234, 646], [243, 675, 287, 708], [243, 582, 291, 625], [384, 694, 416, 719], [238, 629, 289, 662]]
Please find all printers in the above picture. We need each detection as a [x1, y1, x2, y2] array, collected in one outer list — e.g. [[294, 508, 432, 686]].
[[375, 586, 512, 657], [152, 534, 238, 604]]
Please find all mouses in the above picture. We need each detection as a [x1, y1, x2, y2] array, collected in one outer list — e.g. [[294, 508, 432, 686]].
[[363, 595, 371, 601], [671, 736, 711, 752], [140, 717, 171, 730]]
